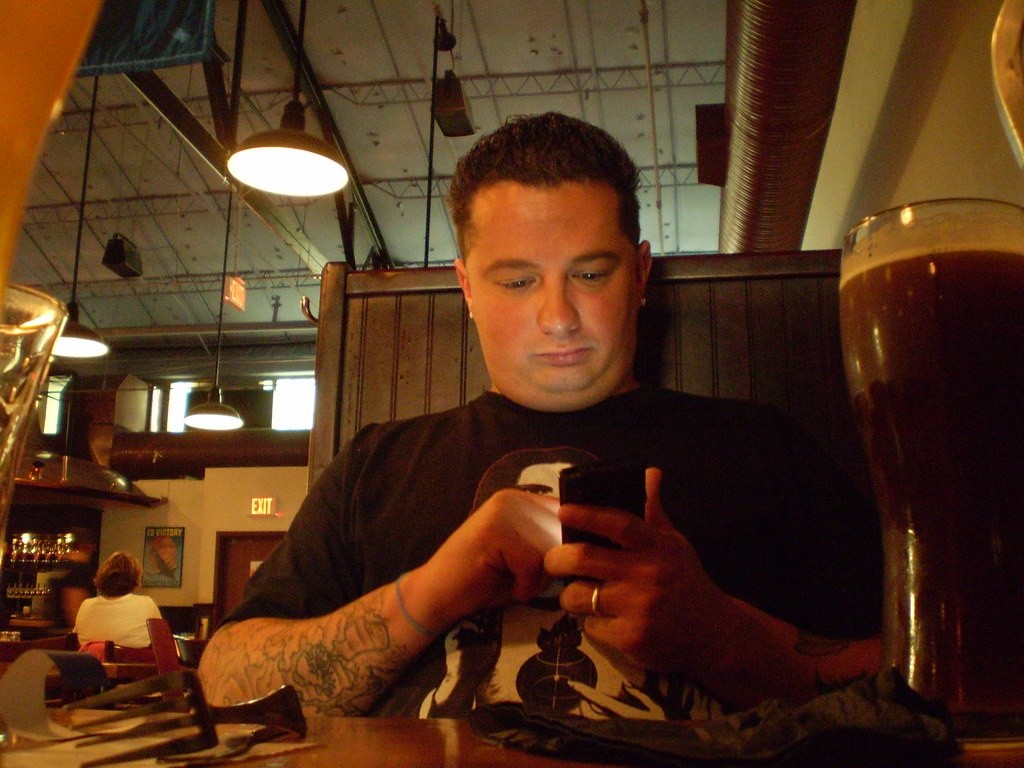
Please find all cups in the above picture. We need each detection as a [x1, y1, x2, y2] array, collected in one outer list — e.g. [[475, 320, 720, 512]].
[[836, 195, 1024, 768]]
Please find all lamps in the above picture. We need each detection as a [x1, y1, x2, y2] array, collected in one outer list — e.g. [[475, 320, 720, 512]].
[[434, 70, 476, 140], [183, 192, 249, 432], [223, 0, 354, 200], [49, 74, 112, 361], [101, 232, 143, 281]]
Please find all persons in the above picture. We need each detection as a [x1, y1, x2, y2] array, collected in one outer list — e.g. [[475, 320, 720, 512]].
[[197, 112, 888, 714], [73, 552, 162, 649]]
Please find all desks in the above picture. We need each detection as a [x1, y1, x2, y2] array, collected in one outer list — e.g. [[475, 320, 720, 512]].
[[0, 706, 1024, 768]]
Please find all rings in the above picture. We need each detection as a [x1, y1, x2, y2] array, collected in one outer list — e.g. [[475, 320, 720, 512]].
[[590, 584, 603, 615]]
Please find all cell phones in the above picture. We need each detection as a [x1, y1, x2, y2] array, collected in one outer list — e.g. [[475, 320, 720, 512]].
[[556, 456, 646, 588]]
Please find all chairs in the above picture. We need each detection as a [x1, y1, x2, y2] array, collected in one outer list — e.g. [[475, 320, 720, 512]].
[[0, 617, 196, 701]]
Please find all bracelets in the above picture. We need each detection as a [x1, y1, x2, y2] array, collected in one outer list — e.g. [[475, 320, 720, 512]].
[[393, 571, 432, 637]]
